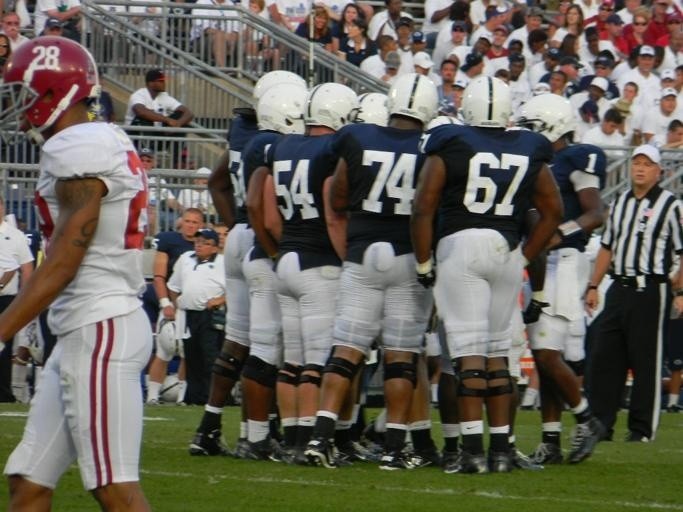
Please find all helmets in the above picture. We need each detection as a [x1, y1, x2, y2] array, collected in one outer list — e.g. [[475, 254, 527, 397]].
[[0, 37, 102, 145], [304, 83, 358, 128], [389, 75, 438, 120], [250, 70, 307, 105], [512, 93, 579, 144], [463, 78, 513, 128], [156, 318, 179, 355], [257, 85, 303, 134], [161, 375, 183, 401], [425, 116, 462, 133], [358, 92, 393, 128]]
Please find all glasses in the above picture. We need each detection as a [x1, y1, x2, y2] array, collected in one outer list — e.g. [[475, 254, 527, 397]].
[[452, 29, 463, 32], [3, 21, 21, 27], [0, 43, 8, 48], [633, 22, 648, 26]]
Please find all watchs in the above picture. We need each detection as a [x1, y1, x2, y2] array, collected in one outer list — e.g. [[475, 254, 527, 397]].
[[1, 284, 4, 288], [673, 290, 682, 298]]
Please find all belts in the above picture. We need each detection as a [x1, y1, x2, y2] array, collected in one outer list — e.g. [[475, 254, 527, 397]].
[[611, 275, 666, 290]]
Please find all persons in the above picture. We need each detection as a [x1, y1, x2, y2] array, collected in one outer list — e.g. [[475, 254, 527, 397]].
[[0, 12, 30, 53], [44, 18, 64, 37], [412, 77, 563, 474], [336, 92, 388, 459], [270, 0, 683, 155], [426, 330, 441, 409], [353, 117, 465, 465], [662, 320, 683, 413], [34, 0, 82, 42], [127, 0, 171, 63], [99, 93, 116, 122], [0, 35, 11, 68], [236, 394, 279, 461], [168, 0, 189, 38], [193, 1, 275, 75], [16, 218, 41, 267], [437, 127, 547, 472], [214, 224, 229, 253], [88, 1, 127, 62], [584, 144, 683, 442], [0, 36, 154, 512], [166, 227, 227, 406], [138, 148, 185, 249], [0, 196, 35, 402], [178, 168, 221, 225], [146, 209, 206, 406], [124, 69, 196, 170], [266, 82, 361, 464], [515, 93, 609, 464], [188, 71, 306, 458], [305, 73, 439, 470], [11, 321, 50, 402], [514, 271, 541, 409], [238, 83, 309, 460], [0, 247, 18, 291]]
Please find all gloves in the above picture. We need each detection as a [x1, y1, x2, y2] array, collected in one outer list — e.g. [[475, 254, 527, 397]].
[[415, 263, 435, 291], [524, 300, 551, 326]]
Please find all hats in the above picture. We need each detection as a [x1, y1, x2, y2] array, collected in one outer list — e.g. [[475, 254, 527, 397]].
[[138, 147, 154, 158], [585, 27, 600, 40], [636, 46, 655, 56], [413, 51, 435, 69], [383, 52, 401, 68], [532, 83, 552, 94], [406, 31, 427, 42], [196, 167, 214, 177], [525, 7, 544, 18], [450, 78, 466, 90], [612, 98, 631, 116], [144, 68, 168, 81], [667, 11, 683, 24], [507, 39, 524, 50], [449, 22, 468, 33], [589, 77, 609, 95], [591, 50, 616, 68], [394, 17, 415, 31], [599, 1, 617, 10], [558, 57, 584, 67], [545, 48, 561, 59], [605, 16, 626, 25], [41, 17, 61, 30], [627, 145, 662, 166], [491, 25, 508, 33], [478, 32, 494, 46], [508, 55, 526, 64], [659, 70, 676, 83], [484, 5, 509, 19], [660, 86, 678, 102], [460, 54, 484, 72], [194, 227, 221, 241]]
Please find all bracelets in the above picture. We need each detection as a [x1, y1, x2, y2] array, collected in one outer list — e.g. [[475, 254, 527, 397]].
[[588, 285, 597, 290]]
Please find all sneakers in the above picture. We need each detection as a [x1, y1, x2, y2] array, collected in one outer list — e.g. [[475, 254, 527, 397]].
[[444, 449, 462, 473], [403, 448, 442, 470], [461, 452, 489, 475], [188, 430, 234, 456], [287, 439, 301, 461], [566, 416, 606, 463], [489, 451, 512, 471], [667, 405, 682, 413], [233, 437, 250, 460], [246, 438, 286, 463], [146, 398, 160, 405], [342, 439, 377, 464], [176, 401, 187, 407], [599, 433, 614, 441], [363, 423, 383, 450], [511, 450, 537, 467], [622, 434, 651, 444], [524, 443, 563, 466], [304, 439, 343, 467], [521, 392, 540, 410], [378, 453, 405, 470]]
